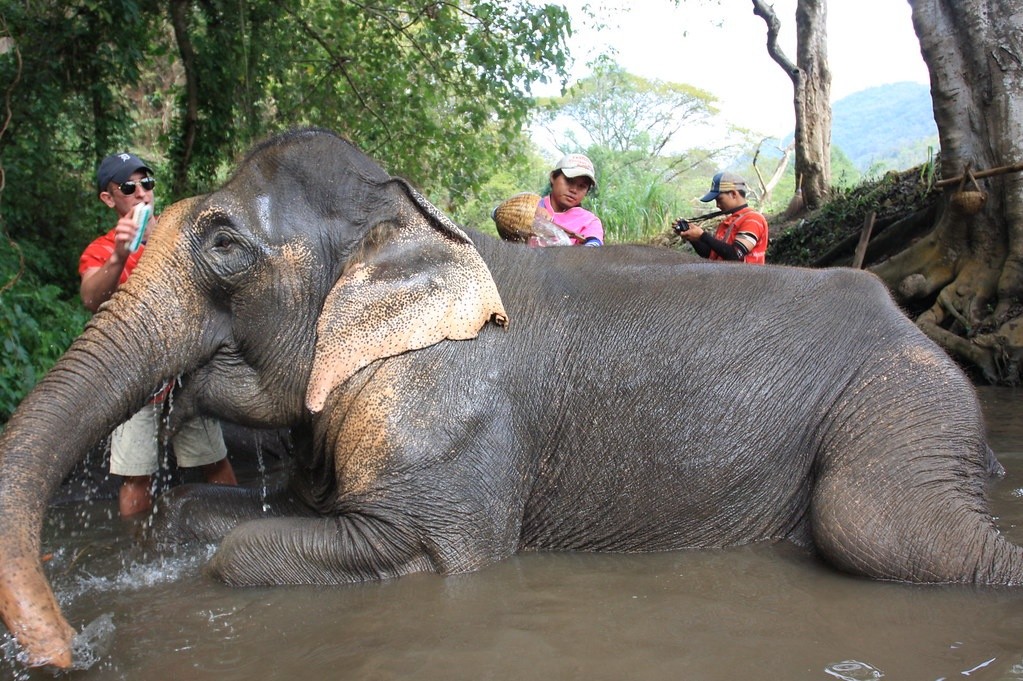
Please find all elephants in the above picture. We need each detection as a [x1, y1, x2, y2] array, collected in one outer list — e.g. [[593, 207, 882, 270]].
[[0, 127, 1023, 667]]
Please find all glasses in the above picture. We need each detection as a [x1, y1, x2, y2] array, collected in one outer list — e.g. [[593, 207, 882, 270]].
[[110, 177, 155, 196]]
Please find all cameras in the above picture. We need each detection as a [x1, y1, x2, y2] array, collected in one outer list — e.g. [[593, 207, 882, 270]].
[[675, 220, 689, 232]]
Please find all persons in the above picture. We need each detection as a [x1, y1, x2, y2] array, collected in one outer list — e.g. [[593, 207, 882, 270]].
[[672, 171, 768, 264], [491, 153, 603, 247], [77, 151, 235, 516]]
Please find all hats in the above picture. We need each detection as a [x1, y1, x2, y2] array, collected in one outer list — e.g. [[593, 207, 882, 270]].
[[549, 154, 595, 186], [97, 152, 154, 194], [700, 173, 748, 202]]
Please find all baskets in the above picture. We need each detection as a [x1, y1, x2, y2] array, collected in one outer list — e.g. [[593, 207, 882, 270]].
[[496, 193, 586, 245]]
